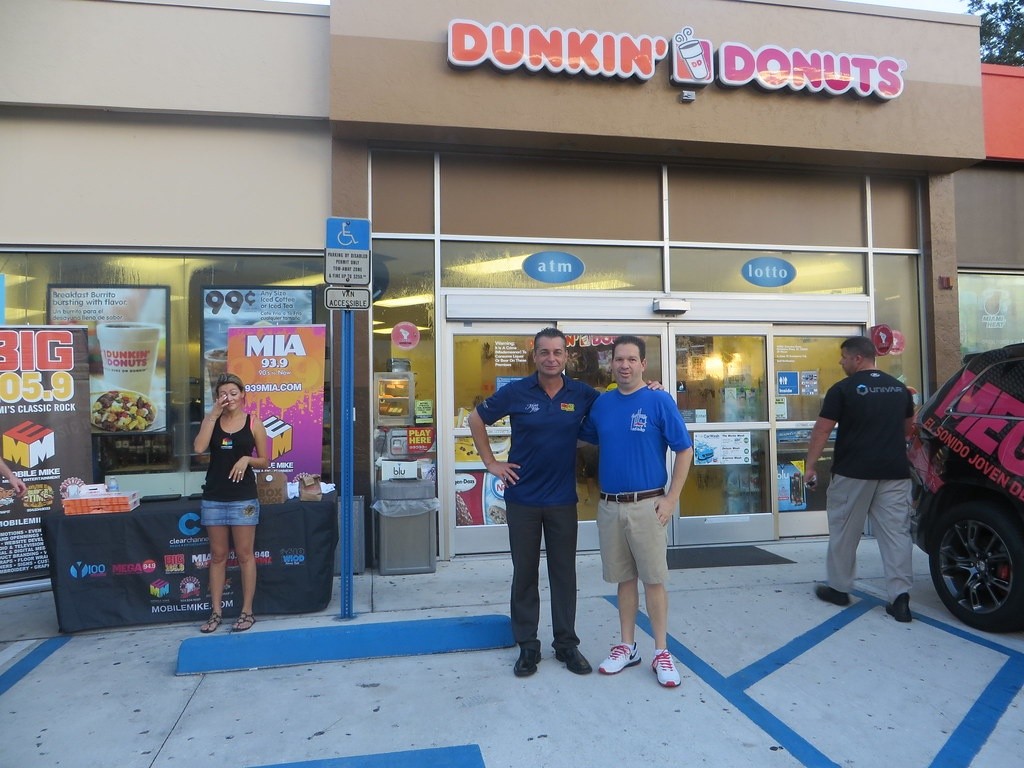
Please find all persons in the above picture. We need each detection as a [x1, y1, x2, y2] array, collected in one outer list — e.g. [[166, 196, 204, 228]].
[[194, 373, 269, 633], [468, 329, 662, 677], [803, 336, 915, 623], [578, 334, 693, 686], [0, 457, 27, 498]]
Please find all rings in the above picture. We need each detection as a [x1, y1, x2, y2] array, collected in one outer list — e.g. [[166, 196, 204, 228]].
[[238, 471, 242, 474]]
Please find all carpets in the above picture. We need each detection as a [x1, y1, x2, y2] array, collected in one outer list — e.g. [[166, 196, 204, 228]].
[[176, 615, 516, 677], [666, 545, 797, 571], [242, 744, 486, 768]]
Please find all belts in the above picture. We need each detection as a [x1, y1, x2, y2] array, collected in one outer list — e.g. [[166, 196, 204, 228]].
[[600, 488, 664, 502]]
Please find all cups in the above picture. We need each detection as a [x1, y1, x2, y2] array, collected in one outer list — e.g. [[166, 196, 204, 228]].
[[97, 322, 161, 397], [204, 348, 228, 404]]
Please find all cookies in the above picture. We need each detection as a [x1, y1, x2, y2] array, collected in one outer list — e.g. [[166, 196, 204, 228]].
[[489, 505, 507, 524]]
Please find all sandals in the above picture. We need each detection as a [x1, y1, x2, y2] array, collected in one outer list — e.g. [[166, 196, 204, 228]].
[[232, 611, 256, 631], [200, 612, 222, 633]]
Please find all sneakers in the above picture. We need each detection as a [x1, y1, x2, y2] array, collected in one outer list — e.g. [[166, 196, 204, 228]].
[[815, 584, 849, 606], [597, 641, 641, 675], [886, 592, 912, 622], [651, 648, 681, 687]]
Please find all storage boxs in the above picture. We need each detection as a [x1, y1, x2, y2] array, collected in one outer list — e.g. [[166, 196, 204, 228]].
[[257, 471, 288, 505], [62, 491, 141, 515]]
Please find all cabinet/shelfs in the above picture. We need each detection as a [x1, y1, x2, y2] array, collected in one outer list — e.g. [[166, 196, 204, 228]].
[[374, 372, 415, 427]]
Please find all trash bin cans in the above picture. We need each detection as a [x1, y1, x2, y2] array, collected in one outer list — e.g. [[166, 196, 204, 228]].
[[375, 478, 437, 573]]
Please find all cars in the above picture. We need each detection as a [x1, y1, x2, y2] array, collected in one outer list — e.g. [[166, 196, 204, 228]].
[[911, 342, 1024, 634]]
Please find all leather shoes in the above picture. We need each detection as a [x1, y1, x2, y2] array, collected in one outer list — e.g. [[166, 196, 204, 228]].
[[513, 645, 542, 676], [555, 644, 592, 674]]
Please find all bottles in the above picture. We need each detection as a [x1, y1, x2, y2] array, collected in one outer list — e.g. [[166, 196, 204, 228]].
[[106, 476, 119, 492]]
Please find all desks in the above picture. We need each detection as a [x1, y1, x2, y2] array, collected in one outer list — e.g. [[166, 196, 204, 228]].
[[42, 490, 340, 632]]
